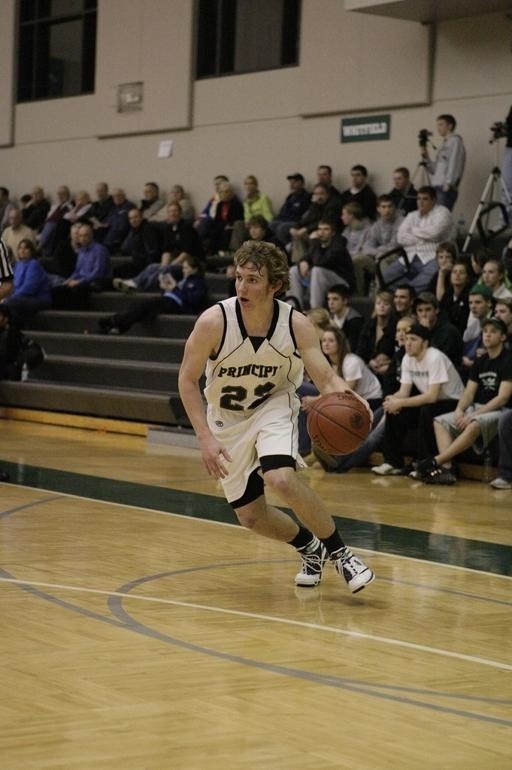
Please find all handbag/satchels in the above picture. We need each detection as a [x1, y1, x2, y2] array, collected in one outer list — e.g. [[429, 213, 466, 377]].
[[10, 337, 46, 371]]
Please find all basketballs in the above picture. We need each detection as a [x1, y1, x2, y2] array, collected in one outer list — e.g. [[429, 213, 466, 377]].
[[307, 395, 368, 455]]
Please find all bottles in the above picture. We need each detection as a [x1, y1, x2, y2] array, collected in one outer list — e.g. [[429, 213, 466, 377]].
[[483, 449, 494, 480], [20, 360, 29, 380]]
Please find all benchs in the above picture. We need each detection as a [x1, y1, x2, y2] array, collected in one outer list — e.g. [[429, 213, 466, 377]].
[[0, 254, 236, 426]]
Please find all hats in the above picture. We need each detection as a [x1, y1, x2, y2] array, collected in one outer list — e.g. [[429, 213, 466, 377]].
[[414, 292, 439, 309], [481, 318, 507, 334], [470, 284, 492, 300]]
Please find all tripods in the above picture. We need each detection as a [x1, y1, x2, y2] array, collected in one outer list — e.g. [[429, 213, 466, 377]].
[[461, 121, 512, 252], [397, 129, 441, 213]]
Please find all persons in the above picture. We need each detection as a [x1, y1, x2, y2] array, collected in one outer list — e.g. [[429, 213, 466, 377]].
[[193, 166, 458, 309], [429, 114, 465, 212], [295, 245, 511, 489], [174, 237, 378, 595], [0, 182, 210, 381]]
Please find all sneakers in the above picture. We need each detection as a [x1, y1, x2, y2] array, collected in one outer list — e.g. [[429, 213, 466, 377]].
[[113, 278, 137, 294], [314, 444, 352, 473], [329, 546, 375, 594], [295, 536, 330, 586], [491, 478, 512, 490], [371, 459, 457, 485]]
